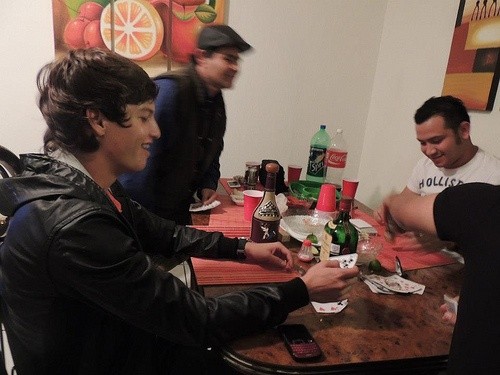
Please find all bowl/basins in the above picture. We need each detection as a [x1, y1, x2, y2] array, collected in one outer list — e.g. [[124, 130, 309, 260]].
[[282, 191, 314, 208], [282, 208, 333, 234]]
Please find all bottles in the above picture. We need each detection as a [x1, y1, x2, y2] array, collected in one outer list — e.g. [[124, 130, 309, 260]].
[[250, 162, 281, 243], [320, 196, 358, 263], [307, 125, 331, 182], [298, 240, 315, 263], [326, 128, 351, 184]]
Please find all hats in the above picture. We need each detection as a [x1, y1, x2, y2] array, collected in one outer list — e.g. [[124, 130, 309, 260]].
[[194, 24, 251, 53]]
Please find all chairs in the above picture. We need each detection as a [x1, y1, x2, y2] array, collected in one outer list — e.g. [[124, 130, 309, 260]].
[[0, 145, 20, 178]]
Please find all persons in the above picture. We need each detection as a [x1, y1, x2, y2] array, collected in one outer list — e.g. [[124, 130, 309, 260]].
[[0, 47, 359, 375], [381, 182, 500, 375], [116, 23, 251, 226], [374, 94, 500, 264]]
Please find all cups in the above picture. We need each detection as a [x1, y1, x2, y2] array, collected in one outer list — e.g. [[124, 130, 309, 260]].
[[357, 232, 382, 261], [341, 178, 359, 199], [243, 189, 265, 222], [314, 184, 337, 221], [245, 162, 261, 185], [288, 164, 302, 184]]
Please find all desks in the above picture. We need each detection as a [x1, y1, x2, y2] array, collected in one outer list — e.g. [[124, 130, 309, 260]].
[[186, 178, 464, 375]]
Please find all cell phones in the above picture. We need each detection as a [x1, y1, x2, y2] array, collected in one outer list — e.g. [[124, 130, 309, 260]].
[[279, 323, 323, 362], [227, 180, 241, 188]]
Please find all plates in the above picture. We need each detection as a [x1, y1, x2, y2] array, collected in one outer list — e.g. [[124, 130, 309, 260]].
[[279, 215, 330, 246]]
